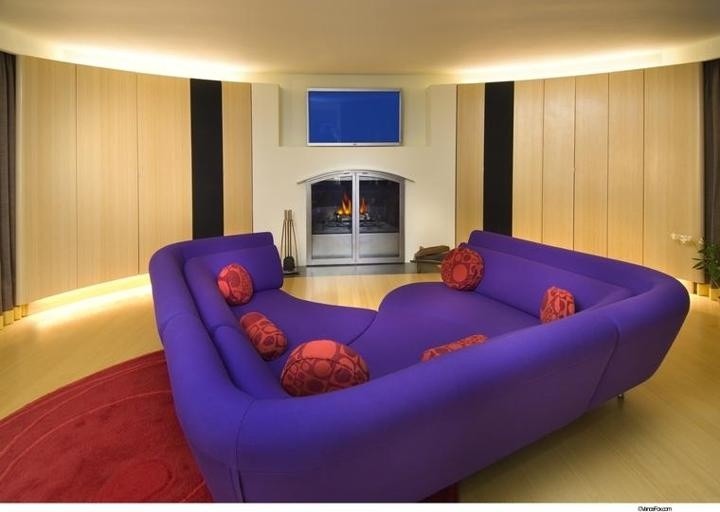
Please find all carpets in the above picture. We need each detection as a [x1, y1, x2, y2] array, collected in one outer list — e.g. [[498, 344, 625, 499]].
[[0, 350, 462, 503]]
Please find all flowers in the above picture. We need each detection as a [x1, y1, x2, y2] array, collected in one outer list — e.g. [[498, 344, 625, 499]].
[[672, 233, 720, 288]]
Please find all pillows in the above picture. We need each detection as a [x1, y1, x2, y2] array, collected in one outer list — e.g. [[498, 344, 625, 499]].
[[240, 311, 288, 355], [217, 264, 256, 306], [442, 248, 484, 290], [281, 339, 370, 397], [420, 334, 489, 361], [540, 286, 574, 322]]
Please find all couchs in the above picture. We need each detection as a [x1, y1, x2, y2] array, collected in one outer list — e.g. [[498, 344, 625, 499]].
[[149, 229, 691, 503]]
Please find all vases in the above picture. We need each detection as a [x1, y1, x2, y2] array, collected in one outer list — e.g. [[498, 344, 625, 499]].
[[709, 287, 720, 300], [697, 283, 711, 296]]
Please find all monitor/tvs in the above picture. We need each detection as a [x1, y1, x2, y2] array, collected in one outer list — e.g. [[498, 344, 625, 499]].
[[306, 87, 402, 147]]
